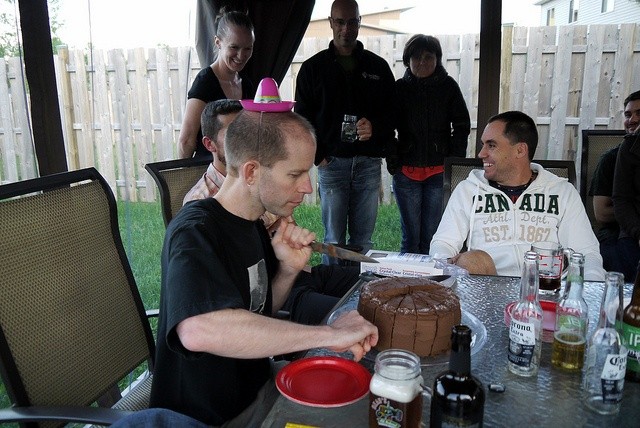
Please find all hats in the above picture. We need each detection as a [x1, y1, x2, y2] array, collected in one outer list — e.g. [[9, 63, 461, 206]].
[[238, 77, 296, 112]]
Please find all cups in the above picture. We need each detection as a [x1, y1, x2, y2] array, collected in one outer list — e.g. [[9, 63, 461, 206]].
[[339, 114, 358, 143], [531, 242, 575, 295]]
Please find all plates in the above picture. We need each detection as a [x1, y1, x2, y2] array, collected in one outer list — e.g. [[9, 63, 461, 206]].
[[327, 301, 488, 367], [429, 265, 458, 287], [275, 356, 371, 407], [505, 300, 581, 342]]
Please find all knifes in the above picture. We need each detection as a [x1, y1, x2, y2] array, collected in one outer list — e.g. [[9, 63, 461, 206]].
[[269, 231, 379, 263], [420, 275, 451, 283]]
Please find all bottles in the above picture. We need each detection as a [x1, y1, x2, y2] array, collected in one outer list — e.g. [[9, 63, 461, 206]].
[[507, 252, 543, 378], [369, 348, 424, 428], [429, 324, 485, 428], [622, 262, 640, 383], [582, 271, 628, 415], [551, 252, 589, 373]]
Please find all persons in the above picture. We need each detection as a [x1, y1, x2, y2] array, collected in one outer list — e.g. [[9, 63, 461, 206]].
[[589, 91, 640, 283], [293, 0, 395, 265], [612, 124, 640, 283], [177, 5, 256, 159], [149, 76, 379, 428], [428, 111, 606, 282], [182, 100, 360, 326], [386, 35, 470, 257]]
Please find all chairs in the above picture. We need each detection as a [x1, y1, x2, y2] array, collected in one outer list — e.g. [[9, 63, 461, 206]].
[[143, 156, 364, 318], [0, 167, 291, 428], [443, 157, 577, 252], [580, 129, 627, 238]]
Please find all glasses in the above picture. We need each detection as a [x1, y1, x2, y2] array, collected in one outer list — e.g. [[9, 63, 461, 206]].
[[331, 17, 361, 25]]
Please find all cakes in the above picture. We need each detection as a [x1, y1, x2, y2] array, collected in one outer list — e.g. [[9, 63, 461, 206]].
[[357, 276, 462, 357]]
